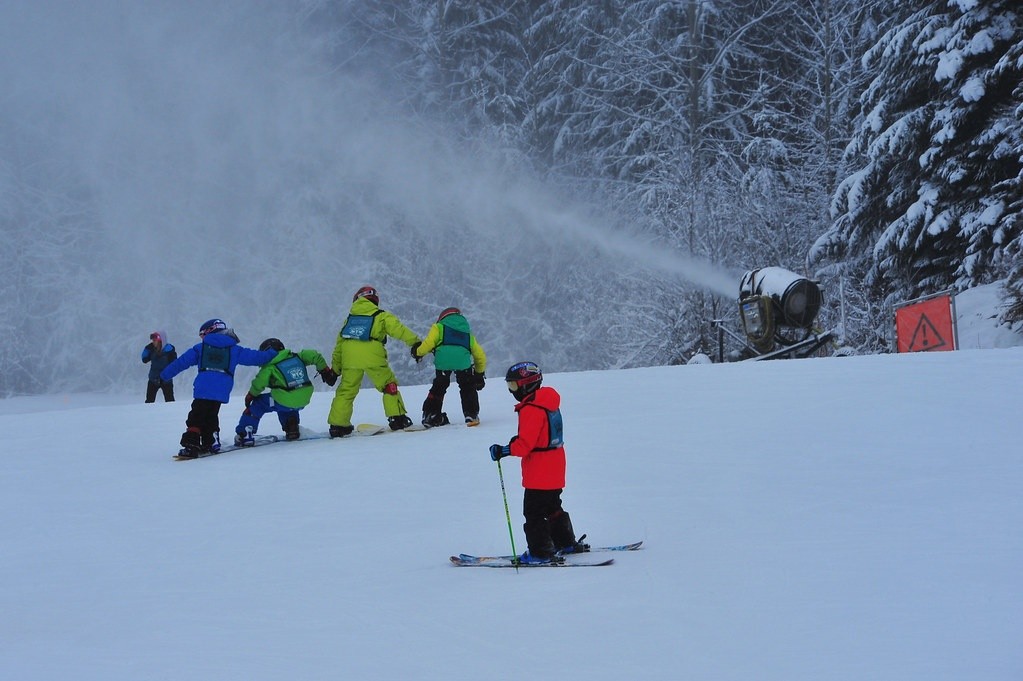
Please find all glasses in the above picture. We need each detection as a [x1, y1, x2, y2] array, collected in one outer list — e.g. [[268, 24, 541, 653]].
[[507, 381, 518, 392]]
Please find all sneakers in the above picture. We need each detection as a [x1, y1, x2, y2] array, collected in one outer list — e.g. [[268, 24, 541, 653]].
[[465, 415, 481, 426], [202, 433, 219, 451], [555, 545, 575, 555], [388, 414, 412, 432], [517, 551, 551, 565], [234, 434, 253, 446], [285, 426, 299, 440], [329, 424, 354, 439], [178, 444, 199, 459], [423, 412, 439, 427]]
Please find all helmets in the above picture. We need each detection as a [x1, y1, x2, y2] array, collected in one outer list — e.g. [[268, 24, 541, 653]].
[[150, 333, 162, 341], [353, 286, 379, 306], [258, 338, 284, 352], [505, 362, 542, 403], [437, 307, 459, 322], [199, 319, 226, 340]]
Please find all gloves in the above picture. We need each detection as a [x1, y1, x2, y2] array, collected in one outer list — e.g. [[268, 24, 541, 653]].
[[272, 342, 281, 351], [245, 392, 253, 408], [471, 370, 486, 390], [151, 374, 164, 387], [489, 444, 511, 461], [411, 343, 423, 360], [321, 369, 338, 386]]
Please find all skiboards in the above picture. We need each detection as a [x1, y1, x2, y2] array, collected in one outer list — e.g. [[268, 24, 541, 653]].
[[173, 417, 480, 460], [447, 540, 644, 567]]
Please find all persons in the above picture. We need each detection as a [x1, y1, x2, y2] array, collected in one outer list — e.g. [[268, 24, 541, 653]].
[[411, 307, 486, 428], [159, 319, 282, 458], [327, 286, 423, 437], [489, 361, 577, 564], [234, 338, 336, 446], [141, 329, 177, 403]]
